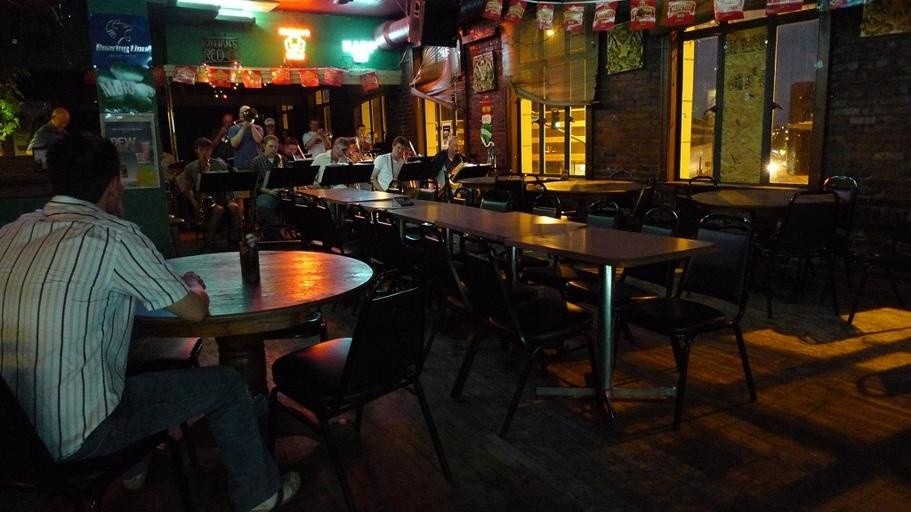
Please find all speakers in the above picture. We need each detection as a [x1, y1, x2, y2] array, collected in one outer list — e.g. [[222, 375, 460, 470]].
[[408, 0, 462, 48]]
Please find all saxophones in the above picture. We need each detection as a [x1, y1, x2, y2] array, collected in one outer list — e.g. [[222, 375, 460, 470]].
[[272, 152, 292, 202]]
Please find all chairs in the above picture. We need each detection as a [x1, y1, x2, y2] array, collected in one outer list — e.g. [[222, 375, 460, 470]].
[[164, 155, 910, 403], [267, 264, 459, 510], [430, 222, 612, 439], [614, 209, 765, 432], [3, 377, 227, 510]]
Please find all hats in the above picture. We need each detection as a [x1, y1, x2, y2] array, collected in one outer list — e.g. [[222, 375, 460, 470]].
[[239, 104, 251, 114], [263, 118, 275, 126]]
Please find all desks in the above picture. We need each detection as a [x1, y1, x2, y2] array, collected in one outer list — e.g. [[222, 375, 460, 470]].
[[129, 246, 377, 407]]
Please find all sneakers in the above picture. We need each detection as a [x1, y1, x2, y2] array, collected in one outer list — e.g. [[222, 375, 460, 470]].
[[244, 472, 302, 512]]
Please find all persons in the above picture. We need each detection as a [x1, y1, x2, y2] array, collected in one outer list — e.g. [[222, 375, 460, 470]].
[[177, 105, 410, 244], [428, 137, 468, 191], [0, 132, 302, 511], [27, 107, 70, 195]]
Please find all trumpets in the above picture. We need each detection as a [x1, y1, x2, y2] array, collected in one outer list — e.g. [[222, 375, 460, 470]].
[[317, 128, 332, 149]]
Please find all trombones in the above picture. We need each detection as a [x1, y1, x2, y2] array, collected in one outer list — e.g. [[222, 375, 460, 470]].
[[195, 155, 216, 225], [234, 107, 258, 128]]
